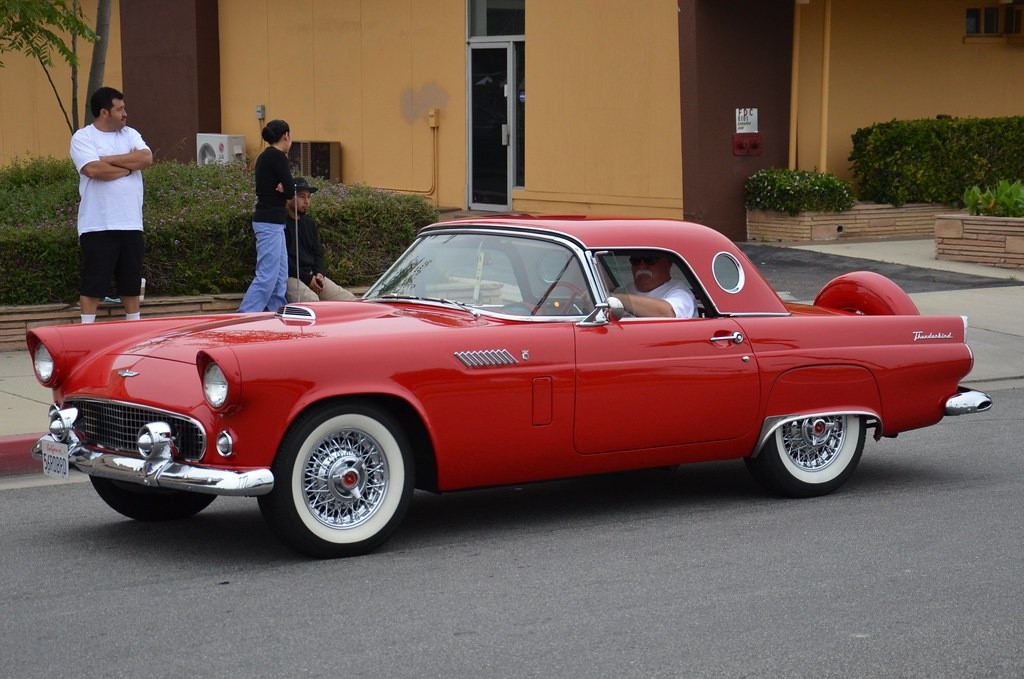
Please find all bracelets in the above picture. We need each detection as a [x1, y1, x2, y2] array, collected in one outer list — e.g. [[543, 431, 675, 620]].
[[127, 169, 133, 176]]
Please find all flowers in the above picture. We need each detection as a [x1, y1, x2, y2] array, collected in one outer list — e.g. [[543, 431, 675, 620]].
[[741, 166, 855, 216]]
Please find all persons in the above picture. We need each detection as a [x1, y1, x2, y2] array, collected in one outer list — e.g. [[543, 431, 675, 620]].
[[231, 120, 296, 313], [70, 86, 154, 323], [581, 248, 701, 318], [284, 177, 357, 304]]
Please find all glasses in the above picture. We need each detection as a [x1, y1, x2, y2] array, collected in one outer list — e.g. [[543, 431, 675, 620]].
[[629, 256, 663, 266]]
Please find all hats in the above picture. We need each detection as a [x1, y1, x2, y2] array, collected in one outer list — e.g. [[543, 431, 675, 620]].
[[294, 176, 319, 194]]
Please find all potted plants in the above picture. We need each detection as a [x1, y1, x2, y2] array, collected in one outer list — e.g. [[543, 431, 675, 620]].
[[935, 178, 1024, 271]]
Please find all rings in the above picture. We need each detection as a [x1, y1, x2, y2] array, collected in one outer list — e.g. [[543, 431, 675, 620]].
[[316, 289, 318, 291]]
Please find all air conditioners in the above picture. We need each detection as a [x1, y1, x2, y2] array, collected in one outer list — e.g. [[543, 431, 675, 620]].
[[196, 133, 246, 169]]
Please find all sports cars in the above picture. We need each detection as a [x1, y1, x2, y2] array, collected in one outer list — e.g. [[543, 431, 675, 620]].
[[24, 215, 995, 562]]
